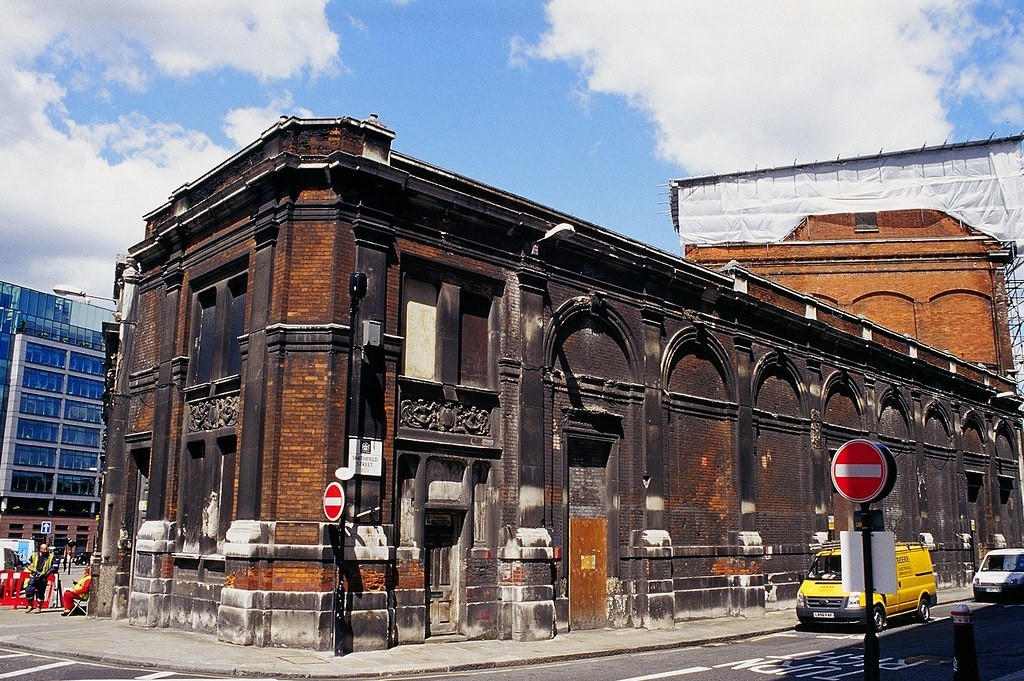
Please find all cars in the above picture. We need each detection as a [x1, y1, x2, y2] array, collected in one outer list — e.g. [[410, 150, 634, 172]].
[[0, 547, 18, 589], [73, 551, 92, 565]]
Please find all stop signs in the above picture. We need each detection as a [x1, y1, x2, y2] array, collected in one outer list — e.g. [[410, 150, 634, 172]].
[[830, 439, 888, 504], [323, 481, 346, 522]]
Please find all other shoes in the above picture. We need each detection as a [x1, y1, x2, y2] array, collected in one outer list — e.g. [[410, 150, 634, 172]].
[[25, 605, 32, 613], [31, 607, 41, 613], [61, 610, 71, 616]]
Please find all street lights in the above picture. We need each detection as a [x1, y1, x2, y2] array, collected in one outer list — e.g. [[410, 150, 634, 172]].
[[333, 269, 371, 656]]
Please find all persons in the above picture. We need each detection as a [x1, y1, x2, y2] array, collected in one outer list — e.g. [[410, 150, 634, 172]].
[[25, 544, 58, 614], [61, 566, 91, 617]]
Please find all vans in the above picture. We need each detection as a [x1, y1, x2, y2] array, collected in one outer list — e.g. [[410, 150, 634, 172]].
[[0, 537, 37, 566], [972, 548, 1024, 602], [795, 535, 938, 633]]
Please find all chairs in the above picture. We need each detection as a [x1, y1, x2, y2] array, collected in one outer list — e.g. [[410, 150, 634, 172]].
[[67, 591, 89, 617]]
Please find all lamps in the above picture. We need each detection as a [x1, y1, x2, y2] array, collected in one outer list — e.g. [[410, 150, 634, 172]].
[[988, 391, 1014, 404], [530, 223, 575, 256]]
